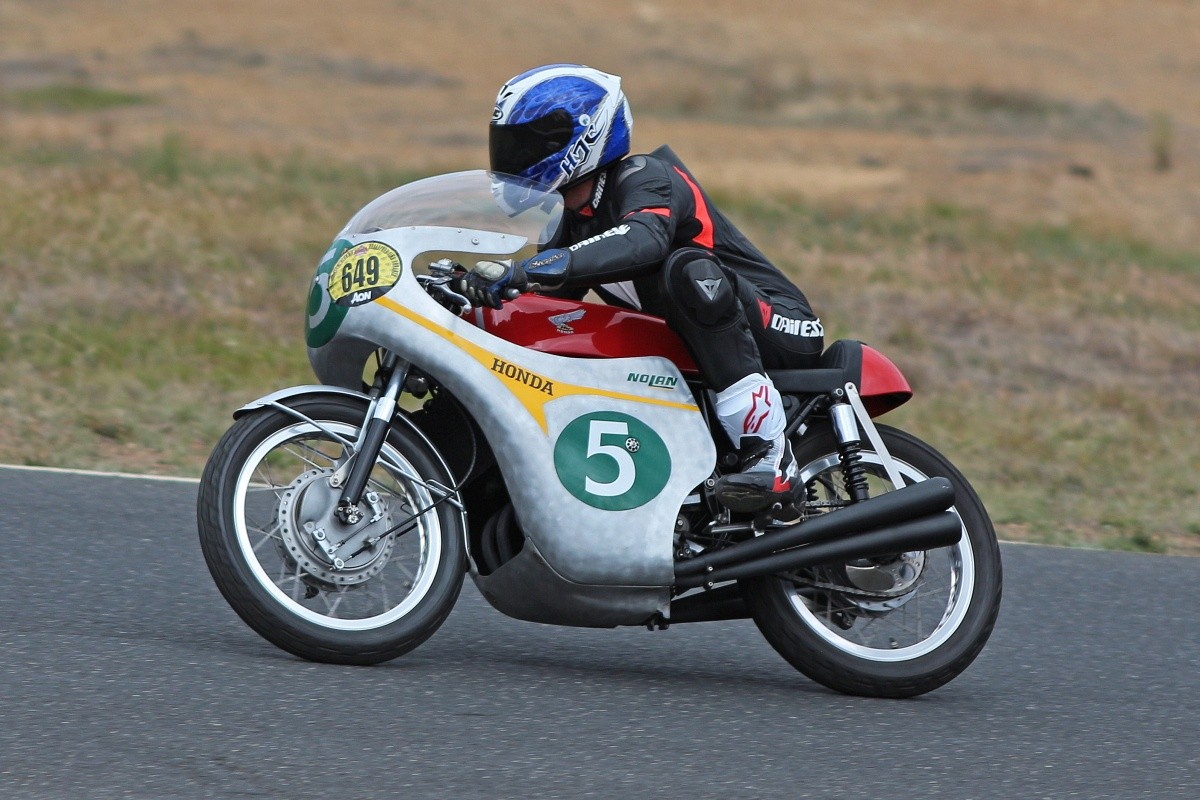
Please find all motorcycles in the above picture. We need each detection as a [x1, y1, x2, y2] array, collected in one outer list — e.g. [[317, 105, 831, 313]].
[[195, 167, 1005, 699]]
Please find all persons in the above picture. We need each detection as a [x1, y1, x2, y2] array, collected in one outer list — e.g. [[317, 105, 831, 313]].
[[462, 63, 826, 511]]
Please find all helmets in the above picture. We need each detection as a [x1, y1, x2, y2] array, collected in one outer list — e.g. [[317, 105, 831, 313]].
[[488, 63, 634, 218]]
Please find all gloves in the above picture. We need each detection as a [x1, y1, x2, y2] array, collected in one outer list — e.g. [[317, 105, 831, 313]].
[[458, 246, 574, 310]]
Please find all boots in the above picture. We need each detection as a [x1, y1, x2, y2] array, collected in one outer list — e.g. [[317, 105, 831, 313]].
[[712, 372, 808, 523]]
